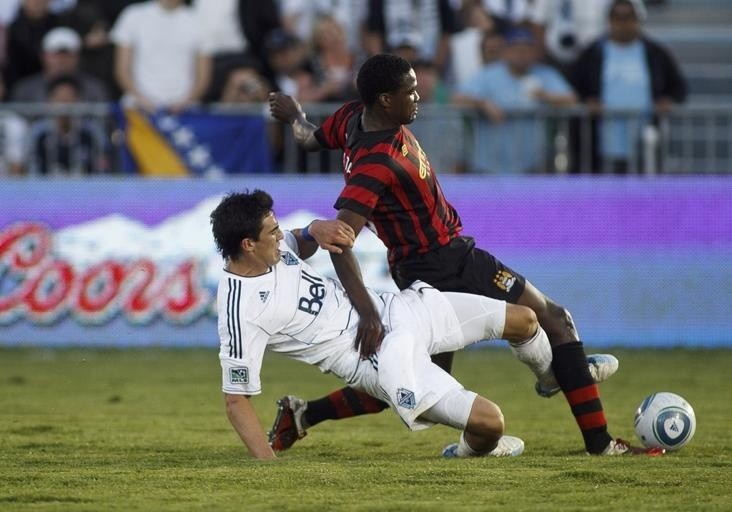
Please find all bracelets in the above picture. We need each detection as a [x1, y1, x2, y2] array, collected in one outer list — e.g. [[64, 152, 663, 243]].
[[302, 226, 315, 241]]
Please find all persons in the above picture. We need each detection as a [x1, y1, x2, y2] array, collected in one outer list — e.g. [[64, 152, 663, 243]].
[[268, 54, 666, 460], [209, 187, 620, 461]]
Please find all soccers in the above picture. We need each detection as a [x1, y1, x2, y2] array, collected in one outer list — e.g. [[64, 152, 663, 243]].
[[633, 392, 697, 451]]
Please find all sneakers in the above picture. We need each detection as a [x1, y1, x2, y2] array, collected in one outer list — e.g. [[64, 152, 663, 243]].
[[535, 353, 619, 398], [442, 435, 524, 458], [267, 395, 309, 451], [588, 439, 666, 458]]
